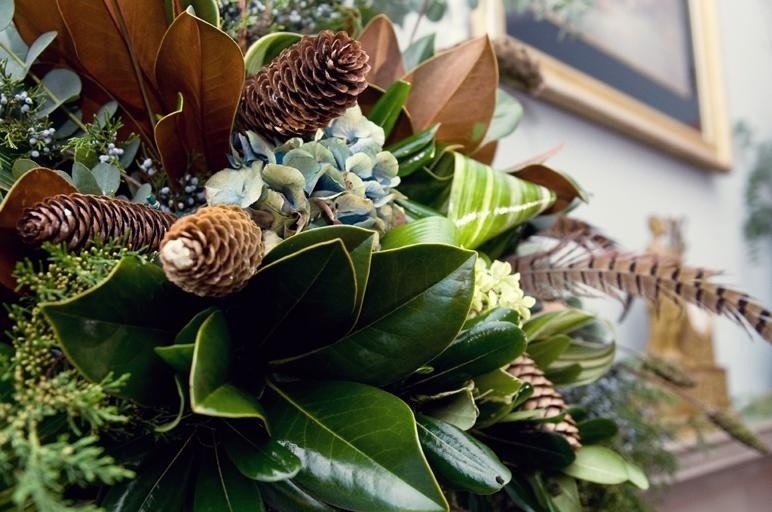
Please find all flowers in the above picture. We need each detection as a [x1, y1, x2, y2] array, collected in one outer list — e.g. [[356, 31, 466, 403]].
[[0, 0, 772, 512]]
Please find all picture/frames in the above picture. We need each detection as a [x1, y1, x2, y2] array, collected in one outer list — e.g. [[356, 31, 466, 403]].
[[471, 0, 733, 173]]
